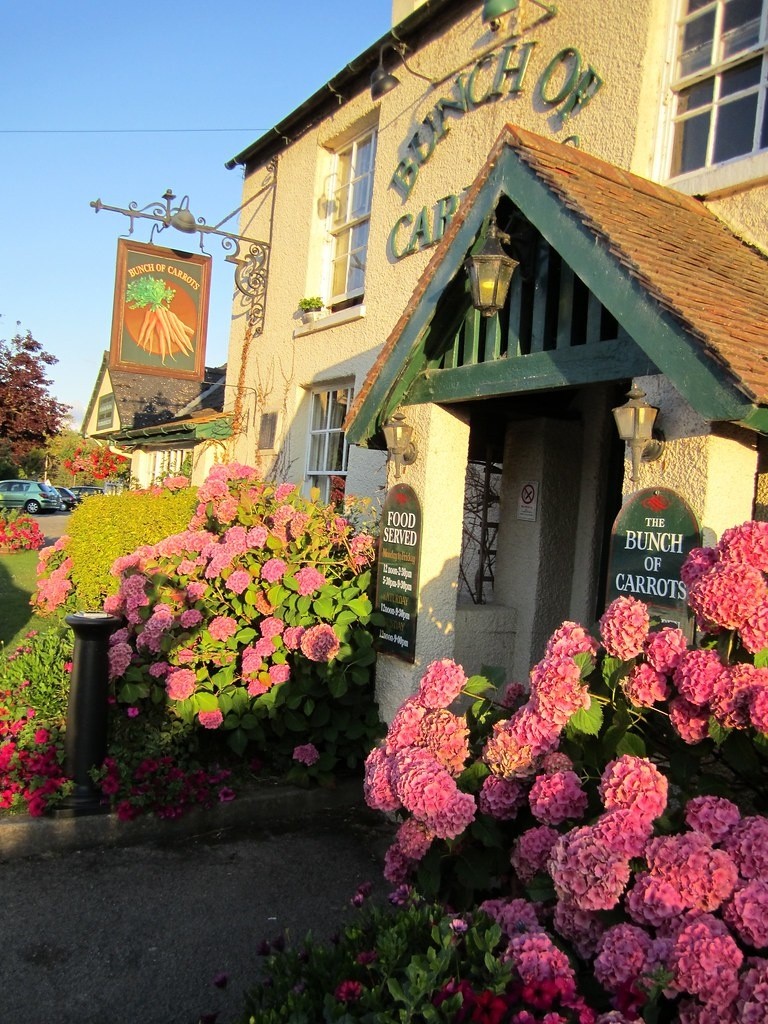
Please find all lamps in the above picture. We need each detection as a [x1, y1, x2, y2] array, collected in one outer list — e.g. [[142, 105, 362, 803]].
[[370, 43, 438, 101], [381, 414, 417, 480], [612, 384, 665, 483], [482, 0, 558, 26], [463, 213, 520, 317]]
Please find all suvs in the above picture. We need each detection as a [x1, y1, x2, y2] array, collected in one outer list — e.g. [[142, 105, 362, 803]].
[[0, 480, 61, 514], [54, 487, 77, 511]]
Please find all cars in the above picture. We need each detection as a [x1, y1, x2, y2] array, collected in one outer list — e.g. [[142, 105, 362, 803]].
[[69, 486, 105, 504]]
